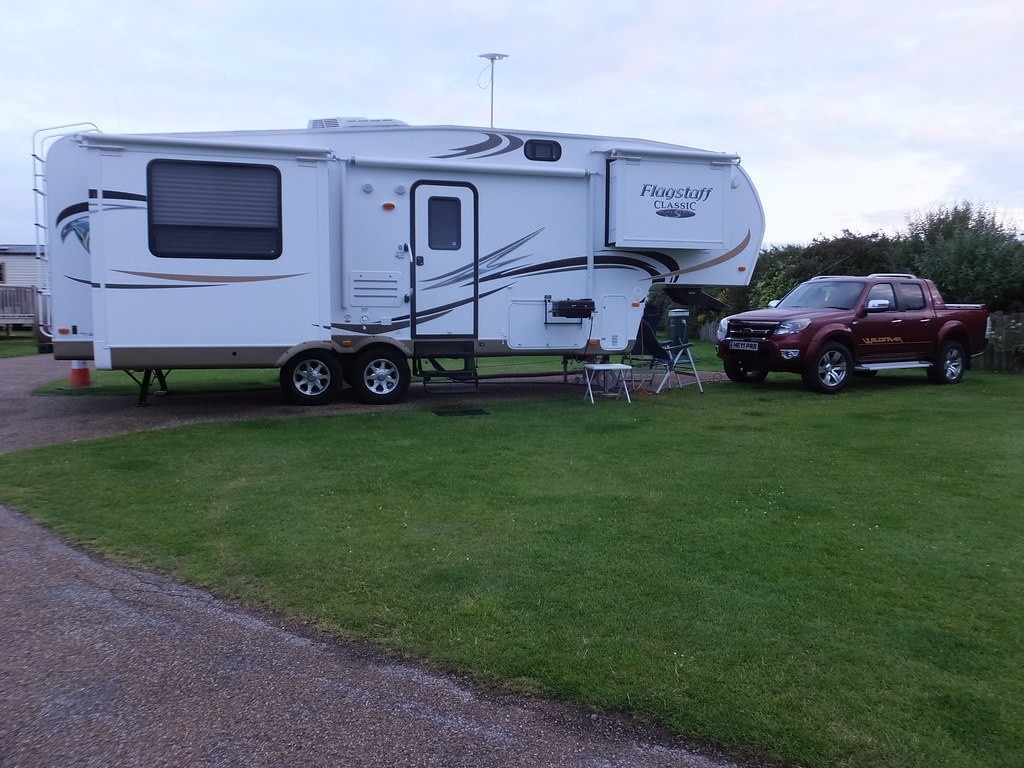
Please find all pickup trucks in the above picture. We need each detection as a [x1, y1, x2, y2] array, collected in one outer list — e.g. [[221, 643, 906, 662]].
[[714, 272, 993, 389]]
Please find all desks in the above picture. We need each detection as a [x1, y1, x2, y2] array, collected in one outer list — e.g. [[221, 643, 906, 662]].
[[582, 364, 632, 404]]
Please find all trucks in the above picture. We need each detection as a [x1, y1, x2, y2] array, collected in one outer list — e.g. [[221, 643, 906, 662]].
[[39, 117, 765, 405]]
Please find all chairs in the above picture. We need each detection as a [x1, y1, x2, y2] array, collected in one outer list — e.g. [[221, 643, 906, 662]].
[[617, 325, 671, 392], [637, 319, 704, 395]]
